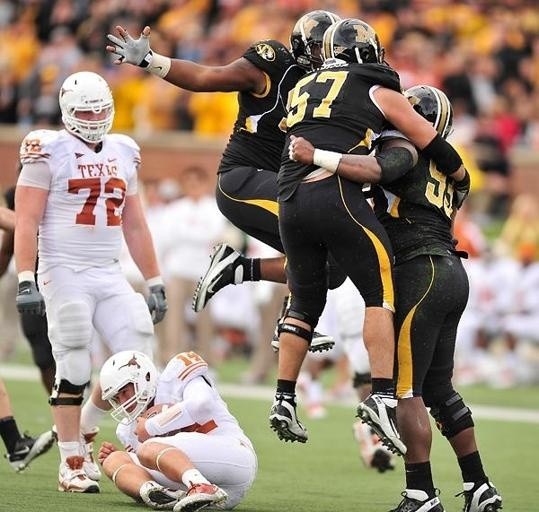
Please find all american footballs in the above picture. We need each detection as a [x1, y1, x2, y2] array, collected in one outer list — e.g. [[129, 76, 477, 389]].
[[144, 405, 180, 436]]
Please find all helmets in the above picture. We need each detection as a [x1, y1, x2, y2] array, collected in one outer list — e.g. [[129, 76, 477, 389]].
[[59, 71, 114, 143], [290, 9, 343, 72], [402, 85, 454, 140], [323, 18, 389, 65], [100, 350, 158, 424]]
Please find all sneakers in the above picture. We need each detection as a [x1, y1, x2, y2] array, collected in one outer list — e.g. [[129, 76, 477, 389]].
[[52, 424, 102, 481], [355, 391, 407, 457], [4, 430, 56, 475], [140, 480, 188, 510], [270, 321, 335, 353], [192, 244, 246, 312], [386, 488, 446, 512], [173, 479, 228, 512], [268, 392, 309, 444], [455, 477, 502, 512], [57, 455, 100, 493]]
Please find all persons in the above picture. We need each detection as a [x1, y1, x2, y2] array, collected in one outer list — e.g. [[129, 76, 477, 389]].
[[12, 70, 170, 492], [1, 2, 539, 393], [103, 8, 505, 508], [0, 128, 80, 477], [91, 347, 258, 508]]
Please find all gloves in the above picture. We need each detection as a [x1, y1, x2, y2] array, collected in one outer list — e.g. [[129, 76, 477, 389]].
[[107, 28, 153, 68], [147, 284, 168, 324], [16, 281, 45, 317]]
